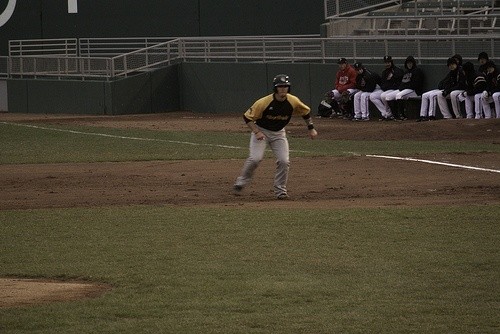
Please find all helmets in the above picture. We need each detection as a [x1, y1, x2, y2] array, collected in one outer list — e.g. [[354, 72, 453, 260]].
[[272, 74, 292, 88]]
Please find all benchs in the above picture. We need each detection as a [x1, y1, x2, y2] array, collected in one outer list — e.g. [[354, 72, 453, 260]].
[[354, 0, 500, 38], [331, 89, 500, 121]]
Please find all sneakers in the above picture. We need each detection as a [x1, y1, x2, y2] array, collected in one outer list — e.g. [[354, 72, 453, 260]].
[[329, 108, 499, 121], [278, 193, 290, 199]]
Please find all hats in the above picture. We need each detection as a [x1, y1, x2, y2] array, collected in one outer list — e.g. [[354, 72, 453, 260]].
[[482, 60, 495, 71], [478, 52, 488, 61], [405, 56, 416, 70], [446, 59, 458, 67], [383, 55, 392, 62], [462, 62, 474, 76], [337, 58, 346, 64], [352, 62, 363, 70]]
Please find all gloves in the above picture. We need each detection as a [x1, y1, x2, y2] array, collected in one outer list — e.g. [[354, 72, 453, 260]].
[[442, 89, 449, 97], [481, 91, 489, 101], [457, 93, 465, 102]]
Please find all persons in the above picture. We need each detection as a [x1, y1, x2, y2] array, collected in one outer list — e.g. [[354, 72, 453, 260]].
[[478, 60, 500, 120], [325, 56, 356, 118], [436, 59, 468, 119], [460, 63, 488, 121], [418, 54, 464, 122], [387, 54, 422, 119], [368, 55, 405, 120], [477, 53, 491, 81], [230, 72, 319, 199], [344, 62, 375, 120]]
